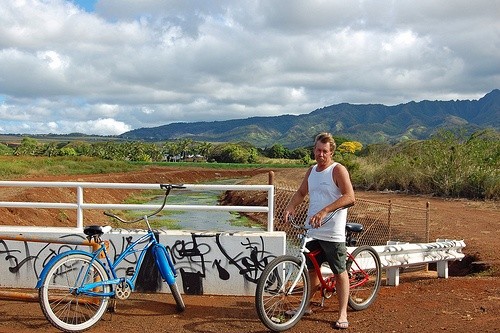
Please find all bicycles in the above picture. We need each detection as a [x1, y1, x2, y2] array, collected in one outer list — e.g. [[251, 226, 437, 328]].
[[255, 201, 382, 333], [33, 184, 187, 333]]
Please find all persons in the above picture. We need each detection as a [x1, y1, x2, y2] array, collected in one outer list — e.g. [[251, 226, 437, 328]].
[[283, 133, 355, 329]]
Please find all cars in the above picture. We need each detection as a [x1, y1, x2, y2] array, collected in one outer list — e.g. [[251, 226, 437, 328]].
[[184, 156, 207, 163]]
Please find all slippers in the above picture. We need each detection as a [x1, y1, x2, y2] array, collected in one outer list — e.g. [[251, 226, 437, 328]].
[[285, 307, 312, 315], [335, 320, 348, 329]]
